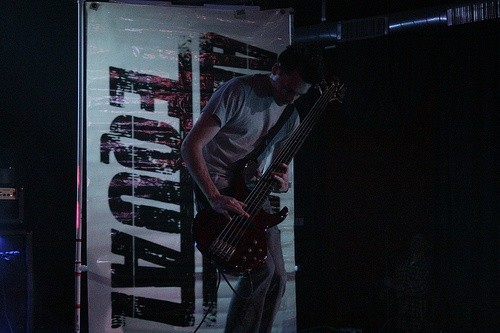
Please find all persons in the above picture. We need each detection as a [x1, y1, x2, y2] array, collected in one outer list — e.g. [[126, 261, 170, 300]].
[[179, 42, 321, 332]]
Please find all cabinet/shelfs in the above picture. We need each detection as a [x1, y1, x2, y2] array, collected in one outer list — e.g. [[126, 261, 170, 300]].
[[0, 229, 34, 333], [0, 182, 27, 226]]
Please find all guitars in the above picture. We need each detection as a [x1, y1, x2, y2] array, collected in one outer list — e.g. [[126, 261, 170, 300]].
[[189, 74, 350, 276]]
